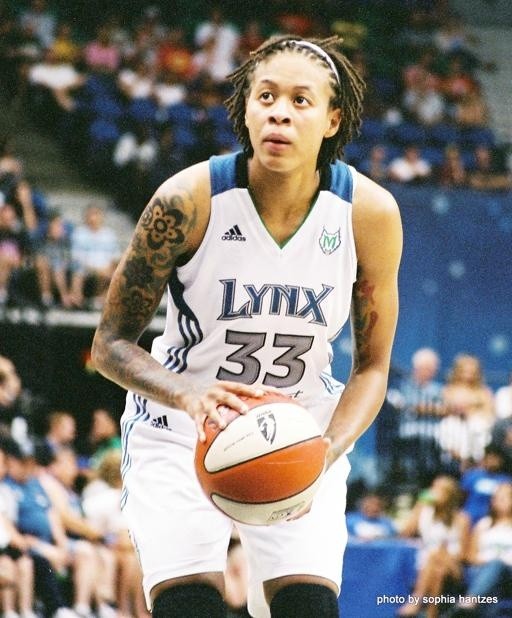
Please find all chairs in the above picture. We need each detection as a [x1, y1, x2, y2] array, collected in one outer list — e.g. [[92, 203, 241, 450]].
[[57, 75, 512, 617]]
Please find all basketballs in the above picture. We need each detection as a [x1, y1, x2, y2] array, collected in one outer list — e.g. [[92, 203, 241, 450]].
[[194, 388, 331, 529]]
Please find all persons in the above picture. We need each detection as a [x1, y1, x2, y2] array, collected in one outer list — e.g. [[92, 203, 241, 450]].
[[0, 0, 511, 616]]
